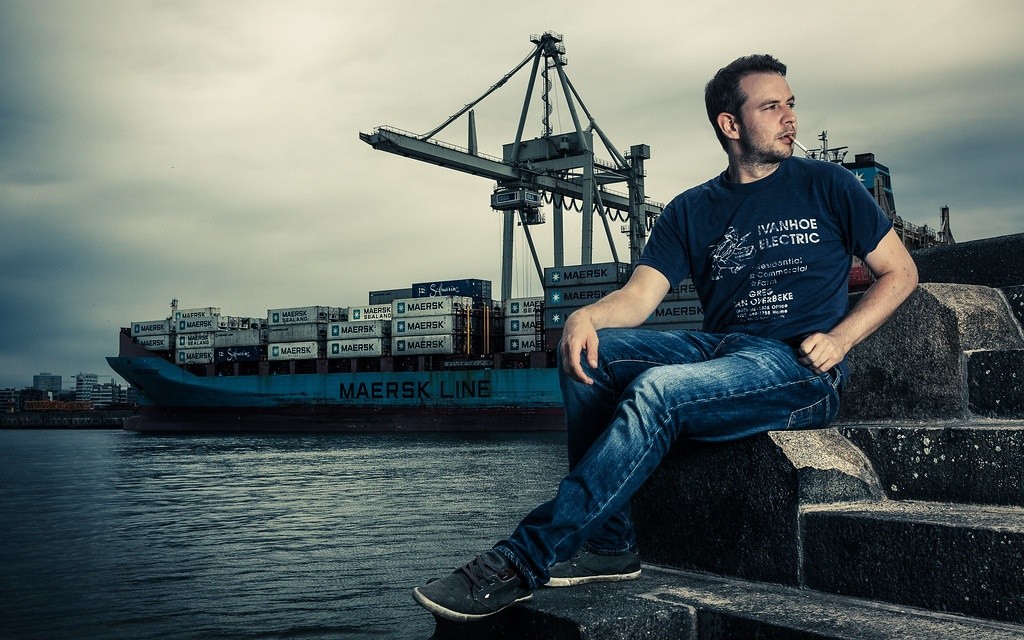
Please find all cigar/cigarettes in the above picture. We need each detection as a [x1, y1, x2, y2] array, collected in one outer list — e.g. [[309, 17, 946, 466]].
[[789, 135, 810, 154]]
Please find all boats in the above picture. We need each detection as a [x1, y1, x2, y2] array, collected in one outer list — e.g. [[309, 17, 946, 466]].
[[103, 25, 956, 431]]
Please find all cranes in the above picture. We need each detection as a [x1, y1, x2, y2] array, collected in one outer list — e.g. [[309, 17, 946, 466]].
[[71, 368, 113, 379]]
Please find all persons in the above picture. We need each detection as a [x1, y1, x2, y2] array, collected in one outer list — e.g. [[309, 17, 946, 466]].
[[412, 54, 919, 623]]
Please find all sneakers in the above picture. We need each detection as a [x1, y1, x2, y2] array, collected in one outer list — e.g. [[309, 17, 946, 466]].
[[412, 548, 534, 622], [543, 546, 642, 587]]
